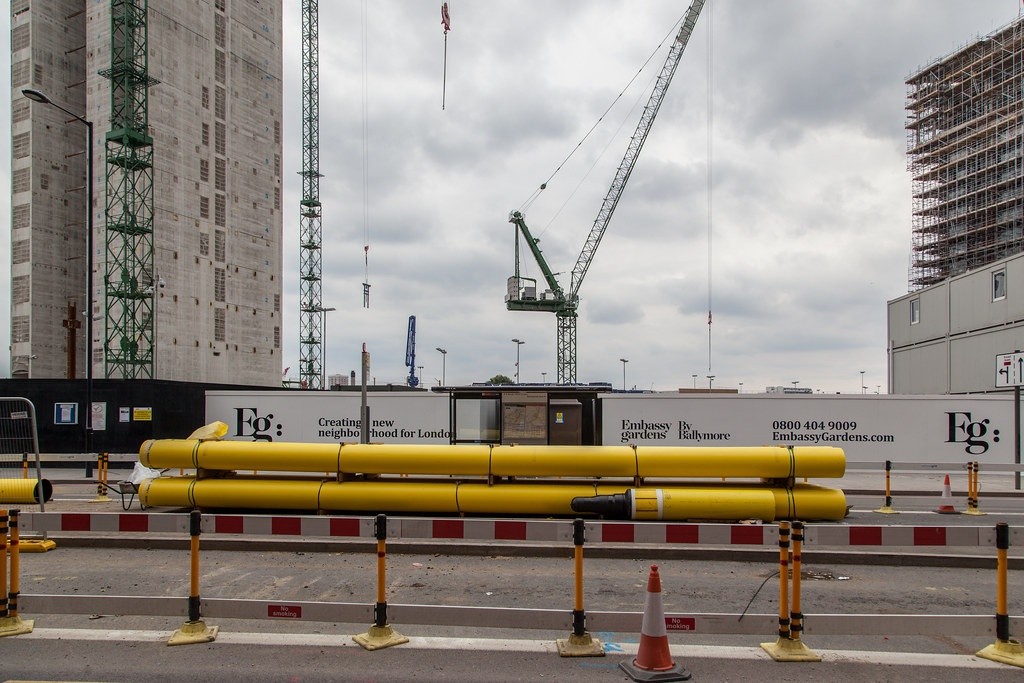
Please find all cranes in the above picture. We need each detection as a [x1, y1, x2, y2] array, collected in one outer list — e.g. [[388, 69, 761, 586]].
[[505, 0, 707, 385]]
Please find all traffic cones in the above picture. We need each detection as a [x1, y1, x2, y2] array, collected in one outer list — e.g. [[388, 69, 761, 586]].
[[618, 564, 691, 683], [932, 473, 961, 514]]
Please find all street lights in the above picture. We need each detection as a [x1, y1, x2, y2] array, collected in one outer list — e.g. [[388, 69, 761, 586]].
[[436, 348, 448, 387], [707, 376, 715, 393], [739, 383, 743, 393], [20, 89, 93, 478], [511, 339, 525, 384], [860, 371, 880, 394], [692, 375, 697, 389], [541, 373, 547, 383], [418, 367, 424, 387], [620, 359, 629, 390], [792, 381, 799, 393]]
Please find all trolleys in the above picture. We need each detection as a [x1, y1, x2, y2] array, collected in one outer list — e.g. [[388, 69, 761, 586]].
[[95, 481, 152, 511]]
